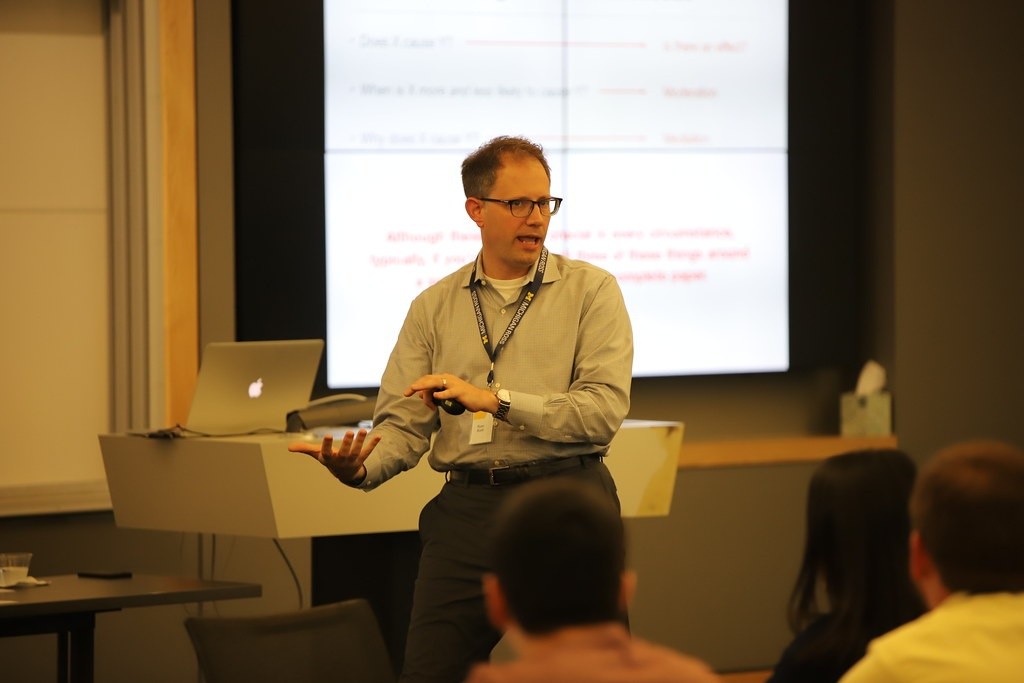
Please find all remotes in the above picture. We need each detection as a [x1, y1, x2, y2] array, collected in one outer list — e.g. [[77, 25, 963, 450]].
[[431, 389, 466, 415]]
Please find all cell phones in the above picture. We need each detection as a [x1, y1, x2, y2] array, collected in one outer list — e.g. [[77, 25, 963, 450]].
[[78, 570, 133, 580]]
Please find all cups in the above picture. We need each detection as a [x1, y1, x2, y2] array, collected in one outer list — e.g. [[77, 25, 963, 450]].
[[0, 552, 33, 587]]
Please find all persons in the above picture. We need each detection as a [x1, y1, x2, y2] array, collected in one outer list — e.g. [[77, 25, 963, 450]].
[[461, 475, 721, 683], [286, 134, 633, 683], [765, 448, 931, 683], [834, 437, 1024, 683]]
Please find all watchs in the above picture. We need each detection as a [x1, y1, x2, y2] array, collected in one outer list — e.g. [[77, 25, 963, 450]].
[[495, 388, 512, 423]]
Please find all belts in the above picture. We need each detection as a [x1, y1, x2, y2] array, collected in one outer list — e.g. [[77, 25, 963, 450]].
[[450, 452, 601, 486]]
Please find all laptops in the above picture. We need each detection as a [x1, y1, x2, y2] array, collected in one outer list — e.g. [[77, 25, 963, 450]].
[[180, 339, 325, 437]]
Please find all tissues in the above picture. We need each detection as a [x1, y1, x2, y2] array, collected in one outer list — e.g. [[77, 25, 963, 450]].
[[839, 359, 893, 438]]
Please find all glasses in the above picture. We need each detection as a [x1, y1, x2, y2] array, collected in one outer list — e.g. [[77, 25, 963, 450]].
[[477, 196, 563, 216]]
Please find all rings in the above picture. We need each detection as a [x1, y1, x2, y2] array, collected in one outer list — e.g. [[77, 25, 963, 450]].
[[442, 377, 447, 387]]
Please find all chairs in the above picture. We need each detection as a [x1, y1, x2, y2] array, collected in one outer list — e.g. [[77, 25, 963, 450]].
[[183, 598, 395, 683]]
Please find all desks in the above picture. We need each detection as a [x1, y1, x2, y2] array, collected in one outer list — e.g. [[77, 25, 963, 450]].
[[101, 418, 685, 675], [0, 572, 263, 683]]
